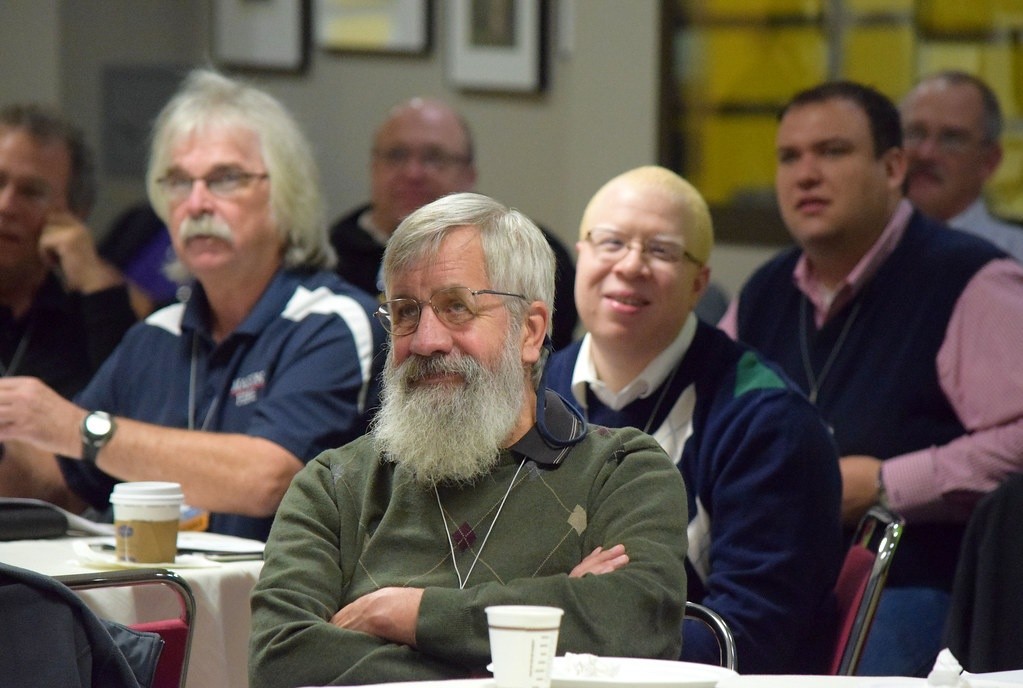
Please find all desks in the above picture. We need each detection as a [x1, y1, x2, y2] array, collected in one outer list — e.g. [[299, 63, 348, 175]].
[[0, 525, 265, 688]]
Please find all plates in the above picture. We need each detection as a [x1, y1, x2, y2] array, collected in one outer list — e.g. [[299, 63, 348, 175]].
[[487, 656, 736, 688]]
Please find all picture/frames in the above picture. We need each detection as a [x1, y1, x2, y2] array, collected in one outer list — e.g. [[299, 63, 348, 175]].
[[309, 0, 434, 56], [438, 0, 552, 96], [209, 0, 311, 76]]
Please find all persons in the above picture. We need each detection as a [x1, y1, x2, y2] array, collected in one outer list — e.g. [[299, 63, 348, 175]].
[[326, 95, 578, 356], [0, 70, 392, 540], [246, 194, 686, 688], [714, 82, 1023, 676], [542, 166, 844, 675], [896, 67, 1023, 264], [0, 102, 194, 513]]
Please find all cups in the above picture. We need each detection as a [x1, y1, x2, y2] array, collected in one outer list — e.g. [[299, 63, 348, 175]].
[[485, 604, 564, 688], [109, 480, 184, 565]]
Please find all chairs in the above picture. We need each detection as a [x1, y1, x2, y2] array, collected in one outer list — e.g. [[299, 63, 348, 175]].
[[823, 506, 905, 676], [49, 567, 197, 688]]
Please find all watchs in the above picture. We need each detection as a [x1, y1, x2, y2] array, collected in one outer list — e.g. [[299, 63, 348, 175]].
[[877, 462, 894, 512], [78, 410, 116, 466]]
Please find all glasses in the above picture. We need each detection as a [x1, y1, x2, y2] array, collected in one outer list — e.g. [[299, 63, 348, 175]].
[[372, 143, 469, 170], [583, 228, 705, 268], [155, 170, 268, 200], [374, 287, 529, 336]]
